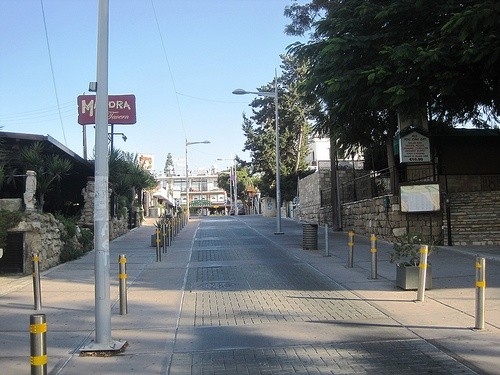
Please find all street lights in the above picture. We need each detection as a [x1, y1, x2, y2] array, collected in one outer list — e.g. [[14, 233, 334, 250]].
[[217, 158, 237, 215], [232, 68, 284, 234], [186, 139, 210, 220]]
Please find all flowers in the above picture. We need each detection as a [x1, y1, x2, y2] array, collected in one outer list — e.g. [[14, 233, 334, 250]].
[[386, 233, 436, 266]]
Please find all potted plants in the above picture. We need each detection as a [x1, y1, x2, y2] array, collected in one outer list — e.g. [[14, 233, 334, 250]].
[[151, 220, 170, 247]]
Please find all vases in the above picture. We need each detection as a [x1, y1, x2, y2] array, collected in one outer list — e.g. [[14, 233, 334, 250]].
[[396, 263, 432, 291]]
